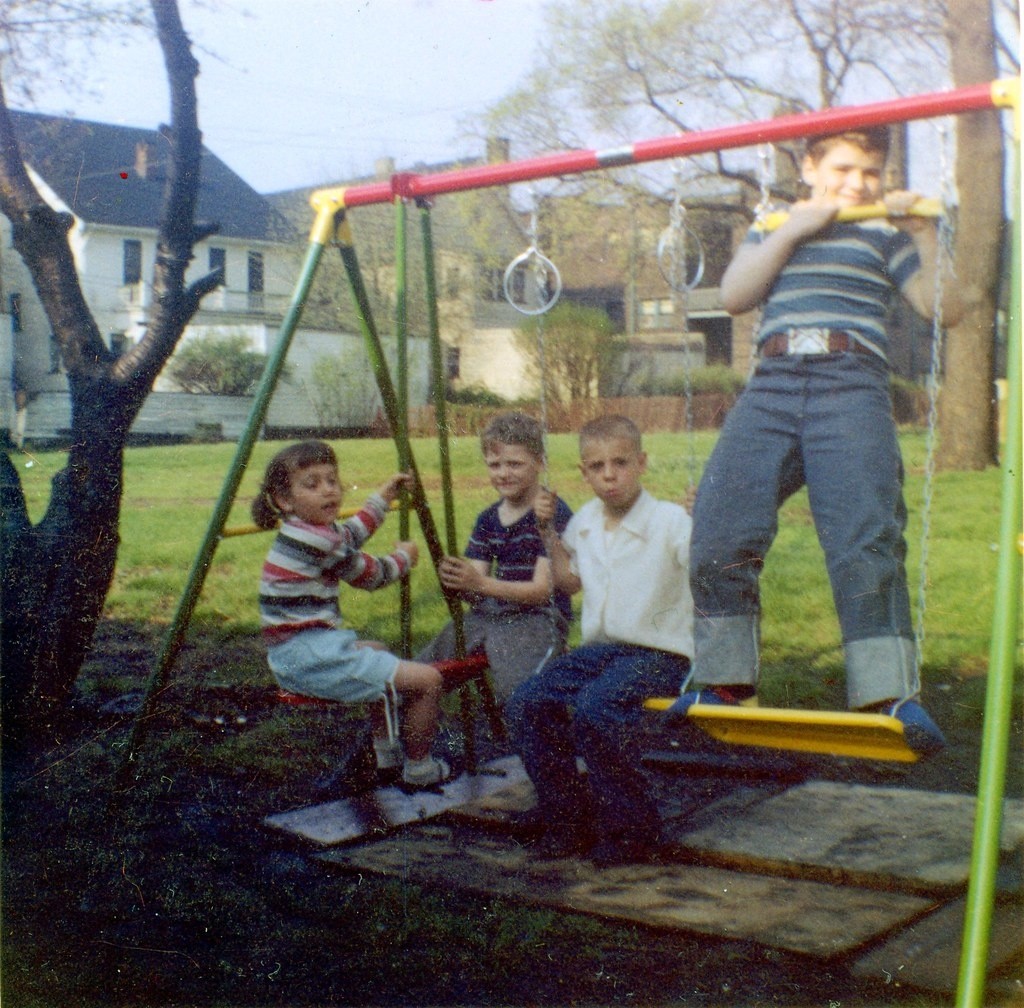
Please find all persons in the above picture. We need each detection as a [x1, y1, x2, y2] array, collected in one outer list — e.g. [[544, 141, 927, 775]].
[[250, 441, 463, 789], [410, 412, 574, 779], [662, 123, 959, 755], [507, 416, 697, 866]]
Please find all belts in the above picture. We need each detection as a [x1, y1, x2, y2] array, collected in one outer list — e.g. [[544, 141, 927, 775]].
[[763, 326, 874, 357]]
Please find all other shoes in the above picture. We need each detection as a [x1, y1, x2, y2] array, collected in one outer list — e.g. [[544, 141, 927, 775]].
[[591, 832, 677, 869], [683, 686, 761, 707], [872, 703, 946, 756], [374, 738, 406, 769], [403, 755, 450, 786], [542, 822, 589, 860]]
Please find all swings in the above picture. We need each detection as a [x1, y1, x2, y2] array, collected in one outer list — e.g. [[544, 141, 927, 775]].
[[643, 118, 950, 765], [519, 157, 685, 735]]
